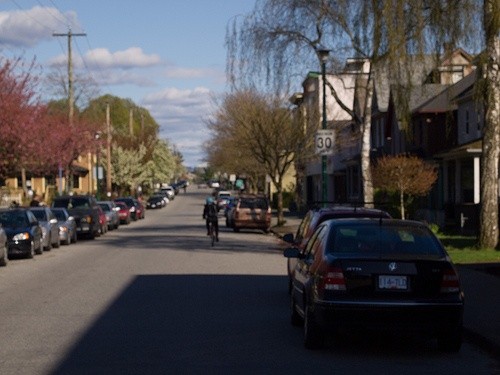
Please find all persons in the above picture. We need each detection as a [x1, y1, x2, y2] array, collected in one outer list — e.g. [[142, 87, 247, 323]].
[[203, 197, 220, 243], [29, 194, 40, 207]]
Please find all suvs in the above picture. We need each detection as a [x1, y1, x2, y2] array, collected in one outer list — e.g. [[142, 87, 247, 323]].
[[282, 206, 398, 297]]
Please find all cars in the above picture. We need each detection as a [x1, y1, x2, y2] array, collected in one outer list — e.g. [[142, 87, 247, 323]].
[[52, 207, 78, 246], [0, 207, 44, 259], [22, 206, 61, 251], [210, 186, 272, 233], [282, 216, 467, 348], [146, 180, 190, 210], [0, 222, 11, 266], [53, 194, 145, 240]]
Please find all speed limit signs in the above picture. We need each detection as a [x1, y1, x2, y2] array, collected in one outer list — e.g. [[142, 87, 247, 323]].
[[315, 129, 333, 155]]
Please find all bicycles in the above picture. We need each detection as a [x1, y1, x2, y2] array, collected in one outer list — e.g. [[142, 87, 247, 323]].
[[202, 215, 218, 247]]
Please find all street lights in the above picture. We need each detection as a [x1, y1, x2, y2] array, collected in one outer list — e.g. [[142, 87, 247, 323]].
[[316, 44, 334, 209]]
